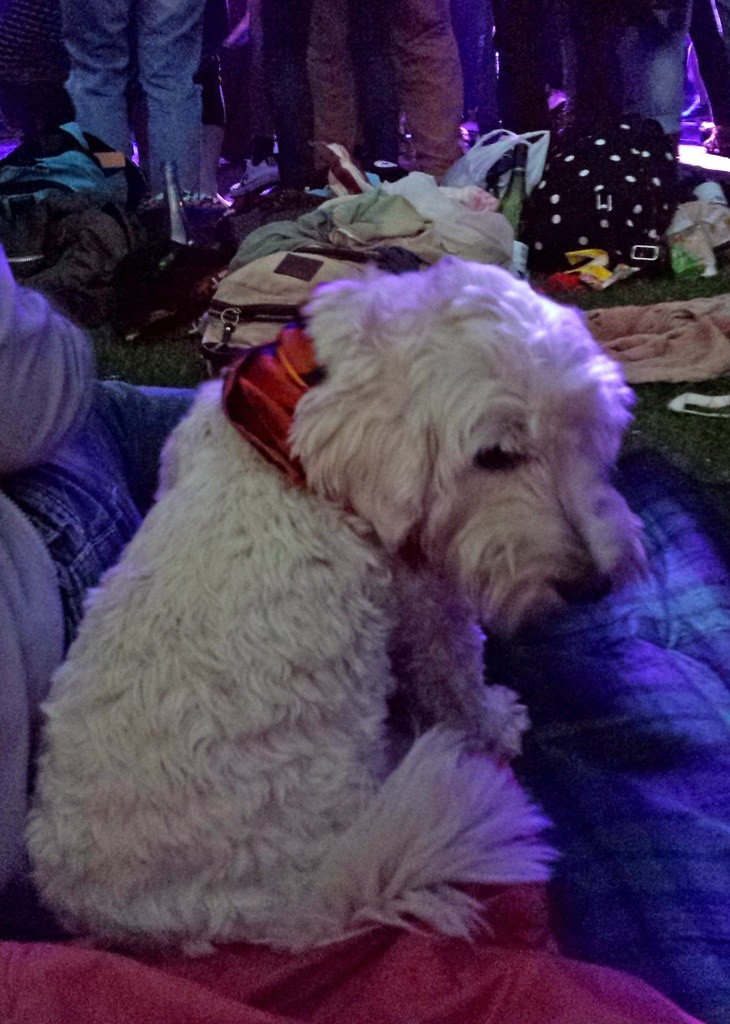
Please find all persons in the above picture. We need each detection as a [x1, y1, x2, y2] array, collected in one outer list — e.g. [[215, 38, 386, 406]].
[[0, 235, 202, 944], [0, 0, 730, 204]]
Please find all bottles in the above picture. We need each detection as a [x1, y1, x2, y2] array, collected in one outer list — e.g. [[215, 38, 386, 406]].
[[494, 143, 532, 279]]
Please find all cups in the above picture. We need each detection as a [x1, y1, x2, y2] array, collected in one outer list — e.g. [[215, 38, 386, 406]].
[[693, 181, 728, 205]]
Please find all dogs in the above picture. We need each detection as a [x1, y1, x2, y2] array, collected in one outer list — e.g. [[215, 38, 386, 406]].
[[19, 255, 653, 960]]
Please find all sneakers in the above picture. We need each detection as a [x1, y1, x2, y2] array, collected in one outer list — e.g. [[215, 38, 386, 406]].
[[229, 157, 279, 196]]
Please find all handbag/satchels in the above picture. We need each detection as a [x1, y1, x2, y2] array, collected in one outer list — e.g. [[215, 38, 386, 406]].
[[383, 107, 679, 280], [198, 247, 360, 362]]
